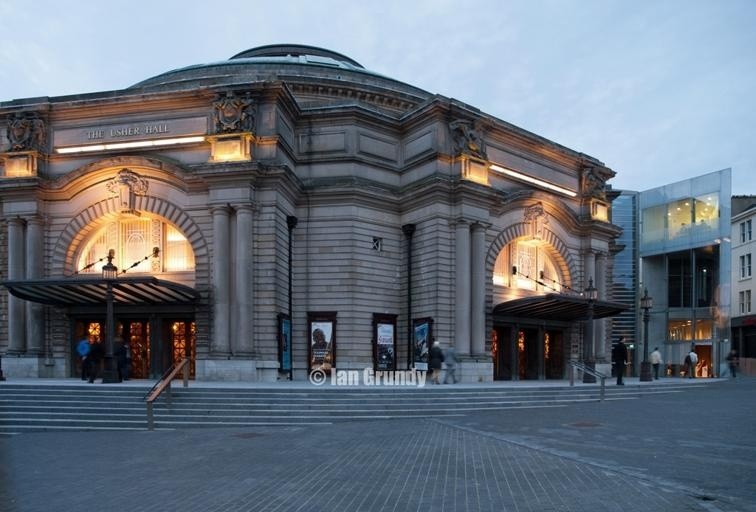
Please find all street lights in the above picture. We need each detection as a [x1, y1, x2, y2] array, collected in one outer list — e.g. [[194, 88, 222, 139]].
[[639, 286, 653, 381], [102, 251, 121, 384], [583, 275, 598, 383], [717, 339, 728, 378]]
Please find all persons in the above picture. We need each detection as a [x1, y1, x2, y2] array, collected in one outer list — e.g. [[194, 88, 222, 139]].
[[689, 349, 698, 378], [611, 335, 629, 387], [76, 332, 131, 383], [683, 351, 691, 378], [585, 166, 606, 198], [460, 118, 487, 157], [441, 343, 462, 385], [650, 347, 663, 380], [312, 328, 331, 367], [428, 340, 445, 385], [725, 349, 739, 378]]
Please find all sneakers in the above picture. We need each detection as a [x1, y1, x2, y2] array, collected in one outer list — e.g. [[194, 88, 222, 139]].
[[443, 382, 448, 384], [618, 382, 624, 385], [453, 380, 457, 384]]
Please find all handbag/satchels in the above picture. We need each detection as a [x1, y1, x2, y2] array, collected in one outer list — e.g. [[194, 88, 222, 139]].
[[685, 356, 690, 365]]
